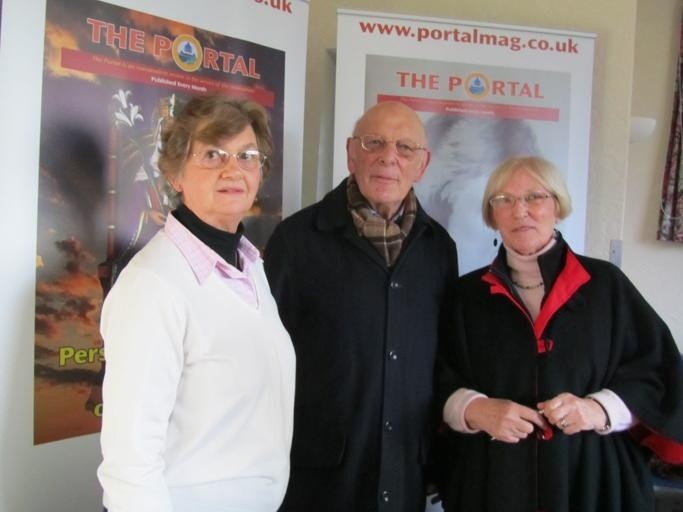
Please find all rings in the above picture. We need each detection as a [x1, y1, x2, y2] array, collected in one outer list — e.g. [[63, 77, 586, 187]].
[[560, 417, 568, 428]]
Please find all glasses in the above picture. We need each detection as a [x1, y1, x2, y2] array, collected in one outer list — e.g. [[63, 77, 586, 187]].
[[189, 149, 267, 171], [354, 135, 426, 155], [489, 192, 554, 208]]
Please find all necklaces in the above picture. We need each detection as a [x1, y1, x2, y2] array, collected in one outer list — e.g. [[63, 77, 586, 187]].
[[510, 278, 544, 289]]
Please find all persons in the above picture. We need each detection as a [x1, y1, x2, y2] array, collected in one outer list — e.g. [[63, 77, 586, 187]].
[[437, 157, 682, 510], [99, 92, 296, 511], [262, 101, 461, 511]]
